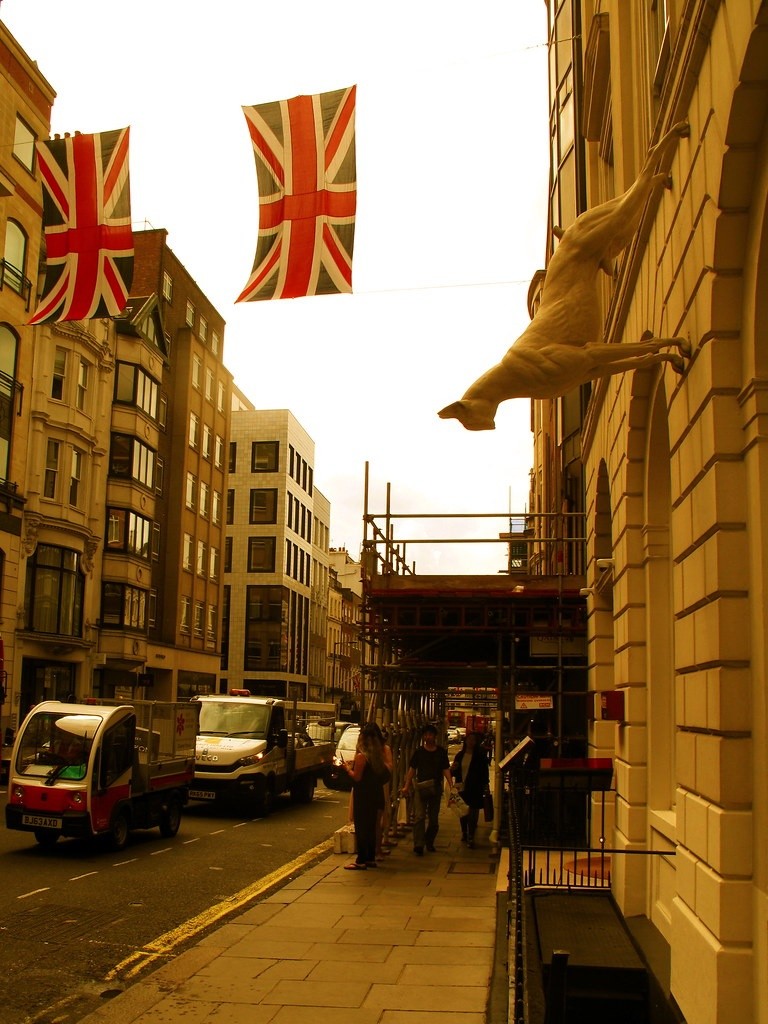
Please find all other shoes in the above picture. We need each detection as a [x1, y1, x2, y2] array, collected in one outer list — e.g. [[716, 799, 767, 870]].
[[413, 846, 423, 857], [426, 842, 435, 852], [375, 855, 384, 861], [467, 836, 474, 847], [462, 833, 467, 841], [489, 762, 491, 766]]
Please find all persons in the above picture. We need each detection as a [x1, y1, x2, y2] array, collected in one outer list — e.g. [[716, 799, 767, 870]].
[[399, 725, 454, 856], [481, 731, 494, 766], [339, 721, 394, 870], [449, 732, 490, 847]]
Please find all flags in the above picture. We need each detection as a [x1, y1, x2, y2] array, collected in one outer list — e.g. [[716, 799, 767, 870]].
[[230, 84, 359, 304], [24, 125, 134, 325]]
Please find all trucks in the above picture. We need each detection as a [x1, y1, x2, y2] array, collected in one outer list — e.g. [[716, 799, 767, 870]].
[[5, 699, 202, 855], [184, 689, 337, 818]]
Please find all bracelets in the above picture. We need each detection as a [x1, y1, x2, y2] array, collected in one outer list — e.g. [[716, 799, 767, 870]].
[[450, 785, 454, 788]]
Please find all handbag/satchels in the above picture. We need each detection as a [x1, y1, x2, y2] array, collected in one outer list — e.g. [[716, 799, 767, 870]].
[[415, 779, 435, 799], [397, 797, 407, 824], [484, 794, 495, 822], [449, 788, 469, 818]]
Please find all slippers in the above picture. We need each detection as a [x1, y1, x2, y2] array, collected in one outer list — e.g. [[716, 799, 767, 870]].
[[344, 863, 366, 870], [365, 860, 377, 868]]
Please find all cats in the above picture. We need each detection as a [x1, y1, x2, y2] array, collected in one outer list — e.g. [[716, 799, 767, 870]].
[[438, 122, 690, 431]]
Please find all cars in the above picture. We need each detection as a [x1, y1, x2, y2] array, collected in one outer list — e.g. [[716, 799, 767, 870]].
[[445, 725, 466, 746], [293, 720, 362, 791]]
